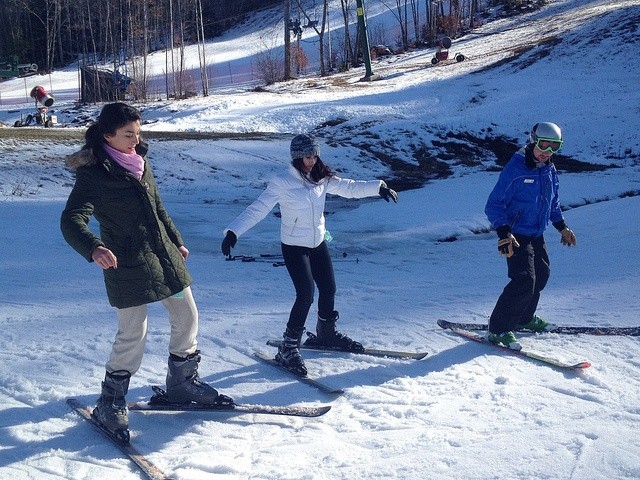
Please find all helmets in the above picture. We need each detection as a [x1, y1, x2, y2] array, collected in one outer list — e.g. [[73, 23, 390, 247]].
[[529, 122, 562, 144]]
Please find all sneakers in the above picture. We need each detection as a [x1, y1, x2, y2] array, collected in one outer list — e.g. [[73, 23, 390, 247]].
[[486, 330, 521, 351], [515, 316, 550, 331]]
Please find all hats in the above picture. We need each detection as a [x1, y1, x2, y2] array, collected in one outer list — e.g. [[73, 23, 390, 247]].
[[290, 134, 320, 159]]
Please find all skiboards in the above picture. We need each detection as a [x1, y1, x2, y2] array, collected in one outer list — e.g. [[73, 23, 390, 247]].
[[254, 340, 428, 393], [66, 397, 332, 480], [437, 318, 640, 369]]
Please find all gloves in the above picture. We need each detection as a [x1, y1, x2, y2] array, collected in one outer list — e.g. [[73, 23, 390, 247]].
[[552, 220, 577, 247], [222, 230, 237, 256], [495, 225, 520, 257], [378, 184, 398, 203]]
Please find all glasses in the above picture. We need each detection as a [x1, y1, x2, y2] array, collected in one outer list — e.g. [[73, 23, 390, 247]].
[[530, 131, 563, 153]]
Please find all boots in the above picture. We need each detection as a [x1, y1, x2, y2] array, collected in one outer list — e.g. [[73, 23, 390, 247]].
[[94, 372, 131, 431], [317, 311, 352, 346], [279, 327, 306, 367], [167, 351, 218, 402]]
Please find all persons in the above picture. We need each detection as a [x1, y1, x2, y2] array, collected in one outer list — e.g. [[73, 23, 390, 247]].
[[485, 122, 577, 349], [60, 102, 219, 438], [221, 134, 398, 376]]
